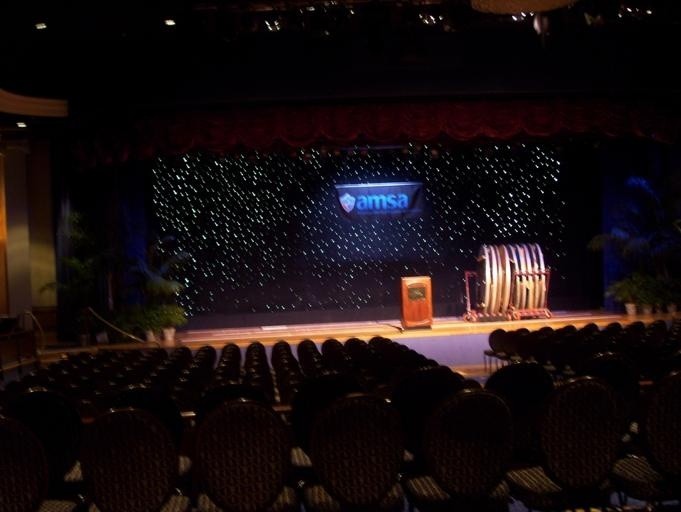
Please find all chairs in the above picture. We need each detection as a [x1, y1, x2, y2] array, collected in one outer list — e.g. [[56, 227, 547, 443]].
[[0, 319, 681, 511]]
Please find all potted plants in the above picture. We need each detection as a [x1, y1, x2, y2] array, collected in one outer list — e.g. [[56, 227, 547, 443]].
[[134, 303, 188, 342], [603, 272, 681, 317]]
